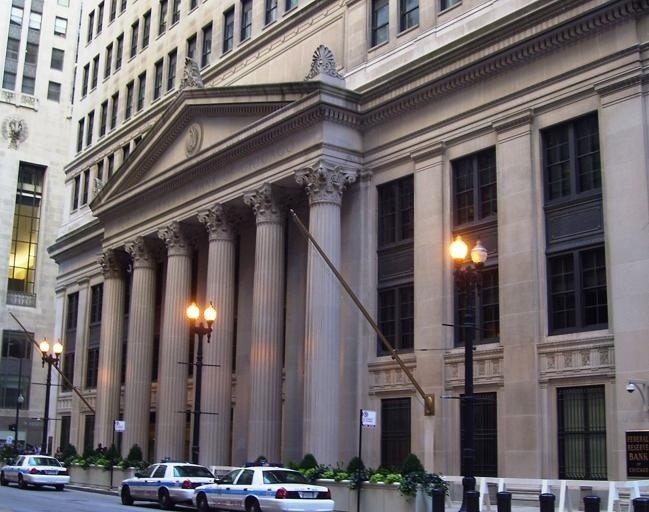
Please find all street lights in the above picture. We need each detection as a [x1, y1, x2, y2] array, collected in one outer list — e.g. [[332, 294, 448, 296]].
[[178, 300, 219, 465], [39, 337, 64, 454], [14, 393, 25, 454], [440, 234, 489, 512]]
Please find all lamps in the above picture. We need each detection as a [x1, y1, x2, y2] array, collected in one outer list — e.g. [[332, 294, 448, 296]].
[[626, 379, 648, 412]]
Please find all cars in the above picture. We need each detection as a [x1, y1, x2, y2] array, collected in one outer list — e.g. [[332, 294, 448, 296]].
[[0, 454, 70, 491], [118, 462, 219, 508], [192, 467, 336, 512]]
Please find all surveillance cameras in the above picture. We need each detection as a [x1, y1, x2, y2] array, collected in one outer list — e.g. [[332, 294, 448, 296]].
[[626, 384, 636, 394]]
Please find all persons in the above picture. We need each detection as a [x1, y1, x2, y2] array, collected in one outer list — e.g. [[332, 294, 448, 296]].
[[0, 439, 44, 467], [95, 443, 104, 455], [55, 447, 64, 462]]
